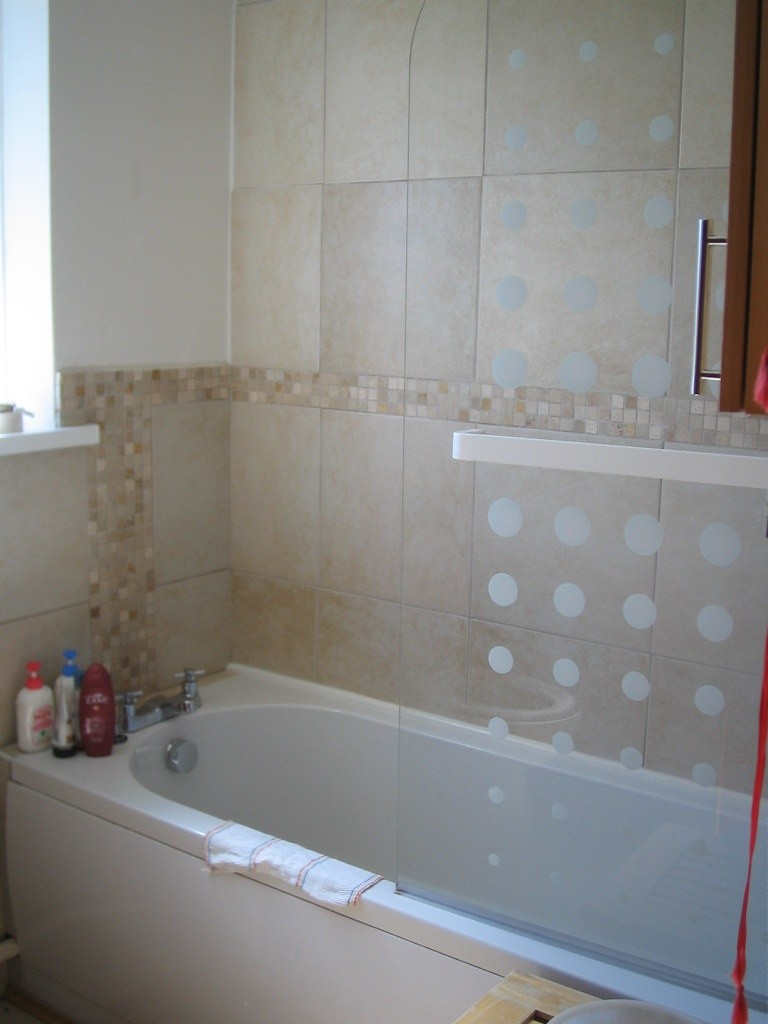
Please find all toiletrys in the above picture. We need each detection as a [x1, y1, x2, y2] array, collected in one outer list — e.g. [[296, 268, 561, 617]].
[[81, 663, 116, 756], [56, 647, 83, 754], [51, 677, 76, 758], [15, 660, 56, 754]]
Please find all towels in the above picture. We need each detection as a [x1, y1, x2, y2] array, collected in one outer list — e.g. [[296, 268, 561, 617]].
[[201, 817, 385, 910]]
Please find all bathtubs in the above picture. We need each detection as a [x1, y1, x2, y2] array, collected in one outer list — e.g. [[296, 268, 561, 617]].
[[0, 660, 768, 1024]]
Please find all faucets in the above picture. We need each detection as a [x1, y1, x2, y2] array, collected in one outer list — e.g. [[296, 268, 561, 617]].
[[162, 698, 195, 714]]
